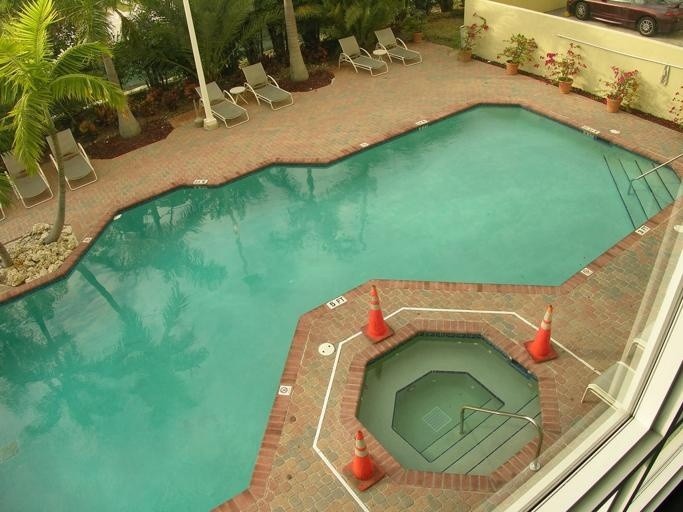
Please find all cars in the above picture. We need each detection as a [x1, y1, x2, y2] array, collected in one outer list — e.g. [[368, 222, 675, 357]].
[[566, 1, 682, 37]]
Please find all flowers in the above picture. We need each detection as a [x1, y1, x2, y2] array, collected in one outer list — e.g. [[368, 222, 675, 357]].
[[544, 38, 589, 82], [455, 10, 490, 52], [668, 86, 682, 130], [595, 60, 643, 113], [494, 31, 543, 68]]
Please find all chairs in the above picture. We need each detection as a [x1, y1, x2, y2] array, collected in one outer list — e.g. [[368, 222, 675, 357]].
[[42, 128, 98, 193], [241, 62, 293, 112], [1, 141, 55, 212], [192, 81, 251, 129], [333, 35, 388, 78], [371, 27, 423, 68]]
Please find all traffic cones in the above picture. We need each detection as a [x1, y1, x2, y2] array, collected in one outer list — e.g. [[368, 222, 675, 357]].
[[525, 304, 559, 364], [361, 284, 395, 345], [342, 430, 385, 492]]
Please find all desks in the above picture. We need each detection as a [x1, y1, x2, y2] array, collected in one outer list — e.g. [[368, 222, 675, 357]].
[[372, 49, 387, 62], [227, 86, 250, 106]]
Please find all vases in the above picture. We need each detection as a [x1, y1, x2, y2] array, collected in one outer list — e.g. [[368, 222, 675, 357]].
[[604, 93, 623, 114], [558, 77, 573, 95], [503, 60, 520, 74], [458, 46, 472, 62]]
